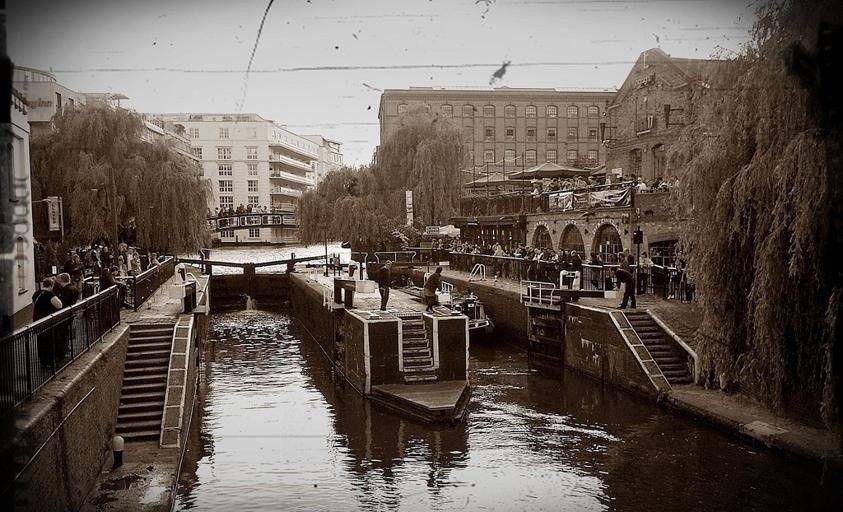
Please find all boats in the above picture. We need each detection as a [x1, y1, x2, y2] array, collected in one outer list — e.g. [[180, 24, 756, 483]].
[[408, 273, 492, 333]]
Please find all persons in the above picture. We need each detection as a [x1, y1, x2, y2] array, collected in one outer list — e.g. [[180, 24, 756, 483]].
[[31, 235, 166, 370], [376, 260, 394, 312], [422, 266, 443, 312], [174, 259, 187, 286], [532, 172, 679, 208], [429, 237, 696, 310], [206, 201, 278, 230]]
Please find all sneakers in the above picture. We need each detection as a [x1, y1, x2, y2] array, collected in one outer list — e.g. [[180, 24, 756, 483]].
[[617, 306, 636, 309]]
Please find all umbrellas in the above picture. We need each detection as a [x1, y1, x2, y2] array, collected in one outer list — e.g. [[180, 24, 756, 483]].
[[507, 158, 592, 183], [463, 170, 532, 194]]
